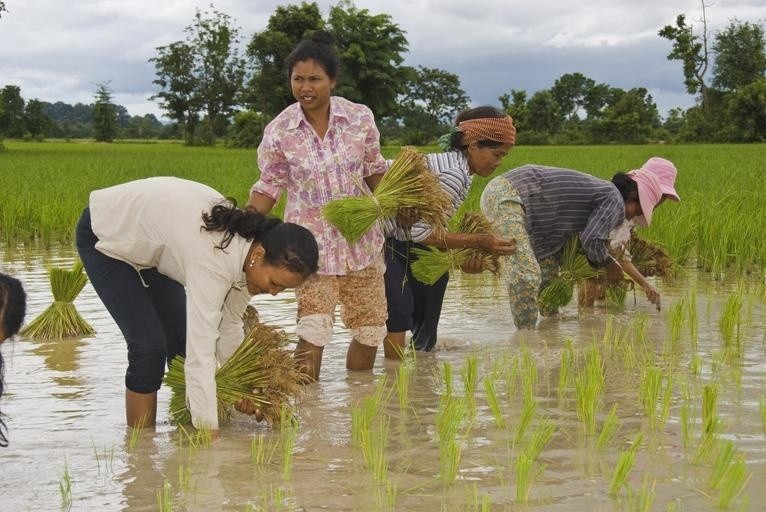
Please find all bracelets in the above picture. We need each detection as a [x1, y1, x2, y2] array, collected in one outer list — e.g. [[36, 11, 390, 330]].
[[463, 232, 467, 247]]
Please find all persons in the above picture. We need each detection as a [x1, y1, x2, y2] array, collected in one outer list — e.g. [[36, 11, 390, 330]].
[[0, 273, 28, 447], [244, 30, 422, 384], [76, 175, 320, 442], [480, 164, 664, 331], [576, 157, 682, 312], [382, 105, 518, 360]]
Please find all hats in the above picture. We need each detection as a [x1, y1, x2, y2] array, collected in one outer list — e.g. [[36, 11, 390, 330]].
[[640, 156, 683, 202], [624, 168, 664, 230]]
